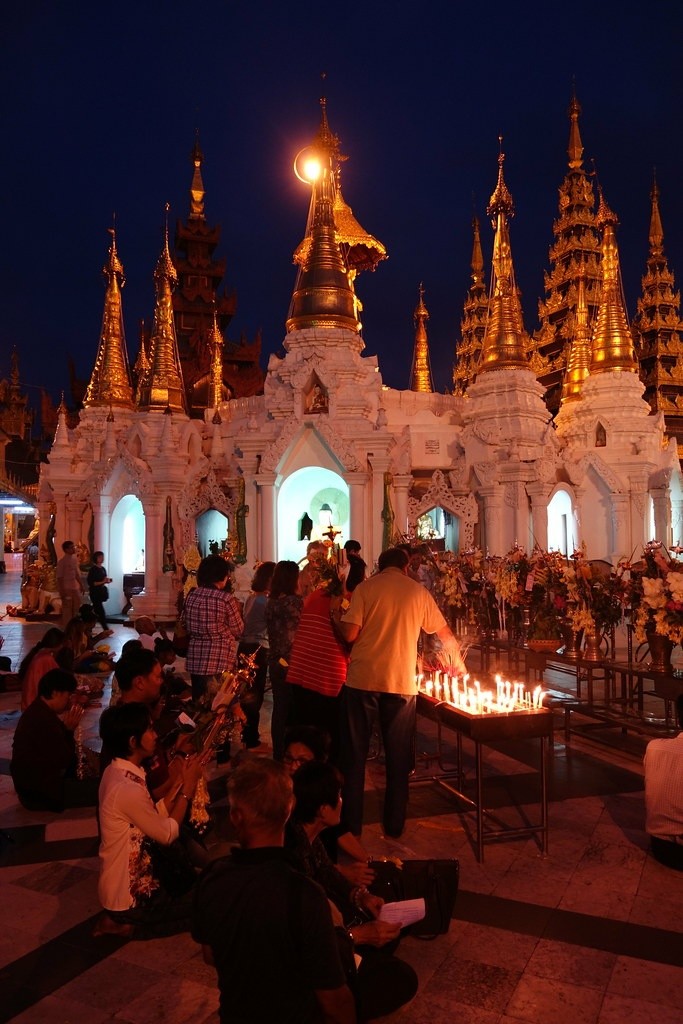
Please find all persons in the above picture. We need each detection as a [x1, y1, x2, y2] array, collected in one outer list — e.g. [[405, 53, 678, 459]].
[[98, 616, 204, 924], [56, 541, 81, 626], [30, 542, 38, 555], [207, 735, 419, 1024], [265, 560, 304, 760], [87, 551, 110, 631], [282, 554, 365, 736], [341, 548, 467, 839], [10, 604, 113, 813], [643, 697, 683, 868], [238, 561, 276, 748], [398, 543, 435, 591], [298, 540, 361, 592], [179, 554, 244, 702]]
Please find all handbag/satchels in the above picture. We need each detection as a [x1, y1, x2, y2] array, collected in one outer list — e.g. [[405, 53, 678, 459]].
[[369, 858, 461, 941]]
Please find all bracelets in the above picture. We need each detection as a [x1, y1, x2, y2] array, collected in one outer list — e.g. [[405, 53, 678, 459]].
[[348, 927, 353, 939], [330, 608, 334, 618], [181, 792, 189, 802], [354, 887, 369, 906], [366, 855, 373, 864]]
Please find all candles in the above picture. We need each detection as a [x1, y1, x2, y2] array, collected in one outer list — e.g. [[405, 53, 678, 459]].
[[416, 667, 546, 714]]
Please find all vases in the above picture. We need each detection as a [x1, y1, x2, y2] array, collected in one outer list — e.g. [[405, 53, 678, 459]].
[[560, 617, 583, 658], [583, 624, 604, 660], [644, 623, 674, 673]]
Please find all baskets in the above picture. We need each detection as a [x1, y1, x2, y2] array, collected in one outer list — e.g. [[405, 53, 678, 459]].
[[528, 640, 561, 654]]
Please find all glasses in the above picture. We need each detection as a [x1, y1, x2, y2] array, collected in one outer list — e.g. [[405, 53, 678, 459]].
[[283, 756, 307, 767]]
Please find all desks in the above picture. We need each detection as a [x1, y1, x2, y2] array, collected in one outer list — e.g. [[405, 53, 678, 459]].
[[417, 689, 556, 862], [467, 635, 683, 741]]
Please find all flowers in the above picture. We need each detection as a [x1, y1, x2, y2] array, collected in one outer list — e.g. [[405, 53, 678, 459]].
[[382, 520, 683, 643]]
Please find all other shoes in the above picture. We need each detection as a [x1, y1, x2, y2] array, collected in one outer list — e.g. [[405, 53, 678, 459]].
[[246, 740, 261, 749]]
